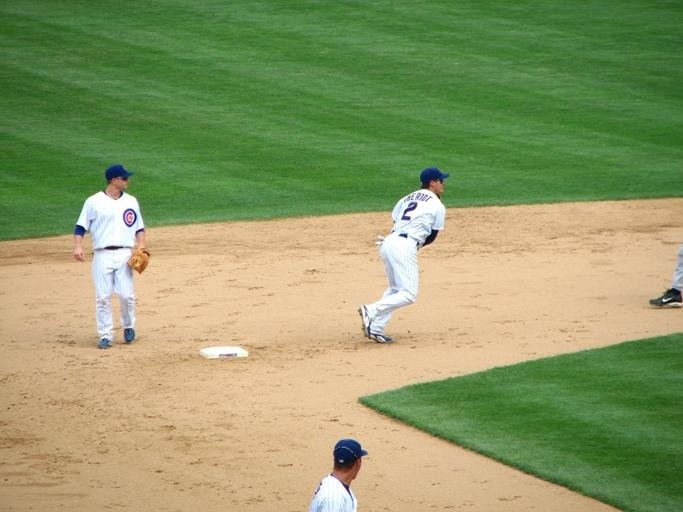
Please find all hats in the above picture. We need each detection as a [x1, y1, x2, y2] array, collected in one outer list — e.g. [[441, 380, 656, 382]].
[[419, 167, 450, 185], [104, 164, 135, 181], [332, 438, 368, 460]]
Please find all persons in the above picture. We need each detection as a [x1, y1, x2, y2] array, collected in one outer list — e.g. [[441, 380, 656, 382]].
[[358, 166, 450, 343], [648, 244, 682, 308], [307, 438, 366, 511], [73, 165, 151, 350]]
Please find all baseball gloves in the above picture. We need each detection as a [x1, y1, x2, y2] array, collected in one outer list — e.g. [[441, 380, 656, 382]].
[[127, 248, 151, 273]]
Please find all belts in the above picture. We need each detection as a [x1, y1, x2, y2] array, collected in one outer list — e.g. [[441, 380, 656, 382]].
[[98, 245, 133, 251], [388, 229, 422, 248]]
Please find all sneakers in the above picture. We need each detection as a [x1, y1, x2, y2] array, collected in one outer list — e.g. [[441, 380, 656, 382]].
[[368, 333, 393, 343], [356, 304, 371, 337], [123, 328, 135, 343], [648, 288, 683, 307], [96, 338, 112, 349]]
